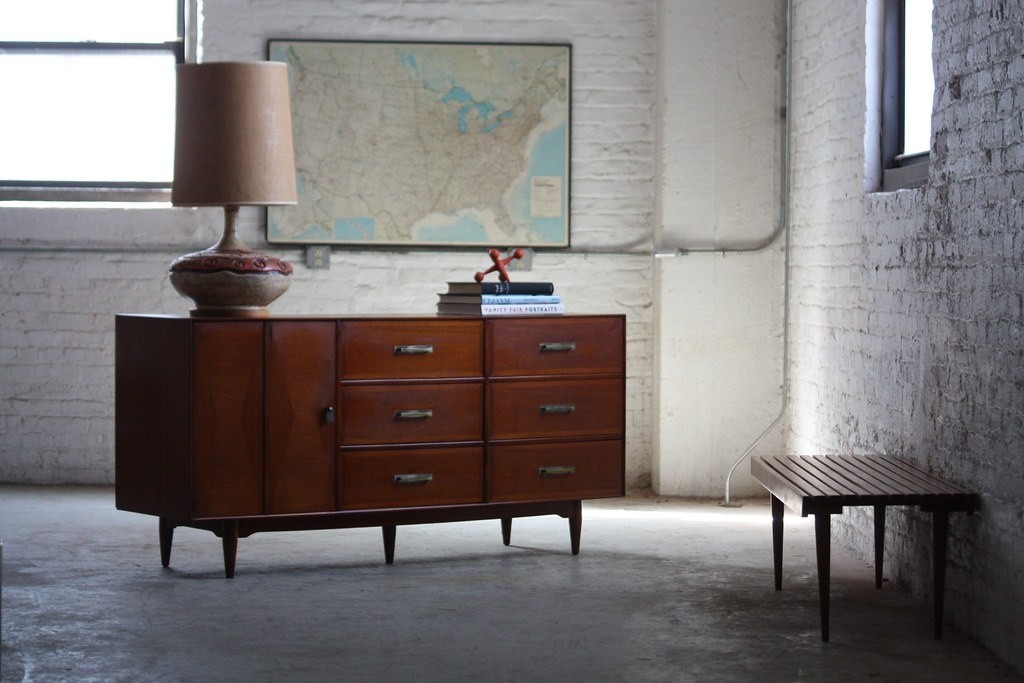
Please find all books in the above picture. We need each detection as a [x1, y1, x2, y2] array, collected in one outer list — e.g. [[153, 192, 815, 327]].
[[437, 293, 560, 304], [435, 304, 565, 315], [447, 282, 554, 295]]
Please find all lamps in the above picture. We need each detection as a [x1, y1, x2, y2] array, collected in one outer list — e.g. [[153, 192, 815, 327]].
[[169, 59, 299, 313]]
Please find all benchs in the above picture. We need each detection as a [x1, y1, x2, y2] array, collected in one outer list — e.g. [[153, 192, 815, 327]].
[[749, 456, 977, 645]]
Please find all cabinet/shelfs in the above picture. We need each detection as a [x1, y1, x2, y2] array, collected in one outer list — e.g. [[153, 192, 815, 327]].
[[116, 314, 628, 579]]
